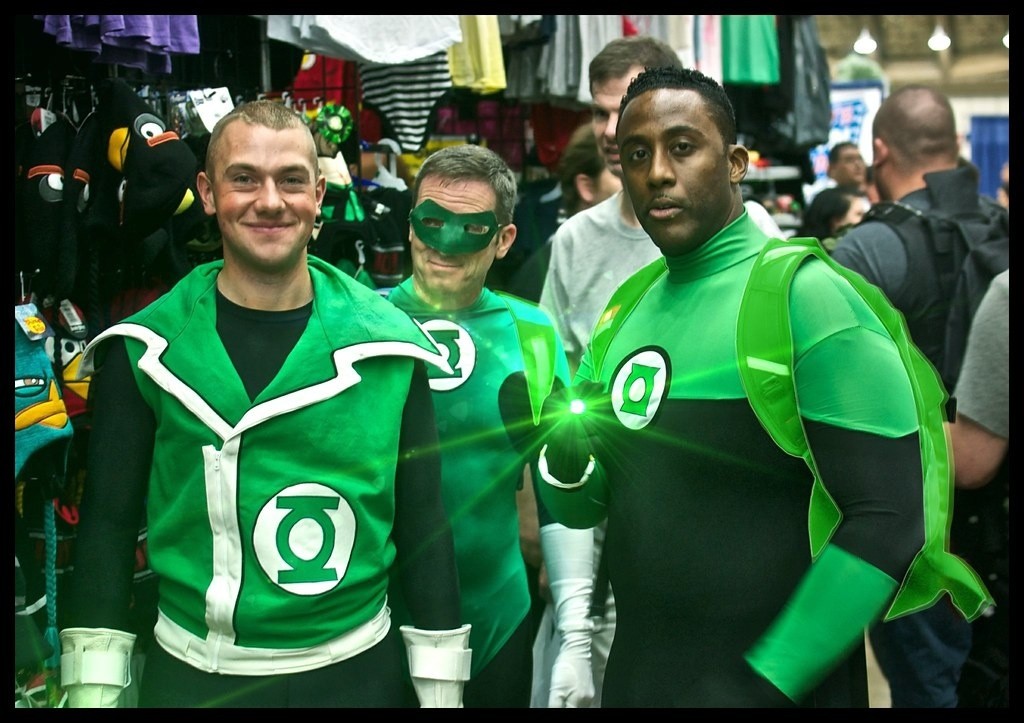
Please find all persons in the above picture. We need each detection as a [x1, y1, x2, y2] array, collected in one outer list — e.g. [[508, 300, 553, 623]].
[[368, 143, 567, 707], [535, 65, 929, 708], [56, 97, 471, 709], [507, 118, 624, 303], [792, 84, 1010, 709], [539, 33, 788, 708]]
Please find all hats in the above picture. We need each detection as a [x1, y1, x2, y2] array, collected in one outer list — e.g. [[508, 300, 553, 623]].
[[13, 70, 207, 649]]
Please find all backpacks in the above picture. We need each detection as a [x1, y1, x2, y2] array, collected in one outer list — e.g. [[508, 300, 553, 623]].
[[861, 199, 1009, 414]]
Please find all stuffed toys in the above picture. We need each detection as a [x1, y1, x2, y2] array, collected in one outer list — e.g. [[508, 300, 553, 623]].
[[28, 87, 197, 241]]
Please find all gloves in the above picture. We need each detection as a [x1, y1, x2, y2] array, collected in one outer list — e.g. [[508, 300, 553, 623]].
[[58, 627, 137, 708], [548, 523, 596, 708], [398, 623, 473, 709]]
